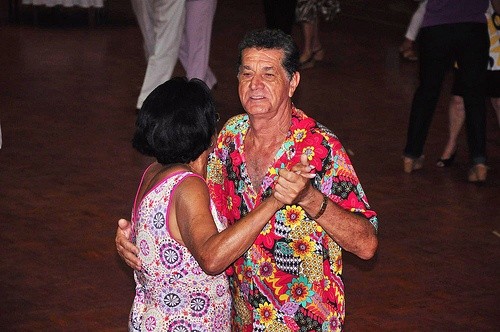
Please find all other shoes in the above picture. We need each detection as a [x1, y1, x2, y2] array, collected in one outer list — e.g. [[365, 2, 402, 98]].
[[135, 109, 141, 114]]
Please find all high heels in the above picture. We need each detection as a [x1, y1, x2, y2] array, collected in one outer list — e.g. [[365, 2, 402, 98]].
[[313, 48, 326, 64], [299, 52, 315, 70], [468, 166, 488, 182], [436, 151, 456, 167], [404, 153, 423, 173]]
